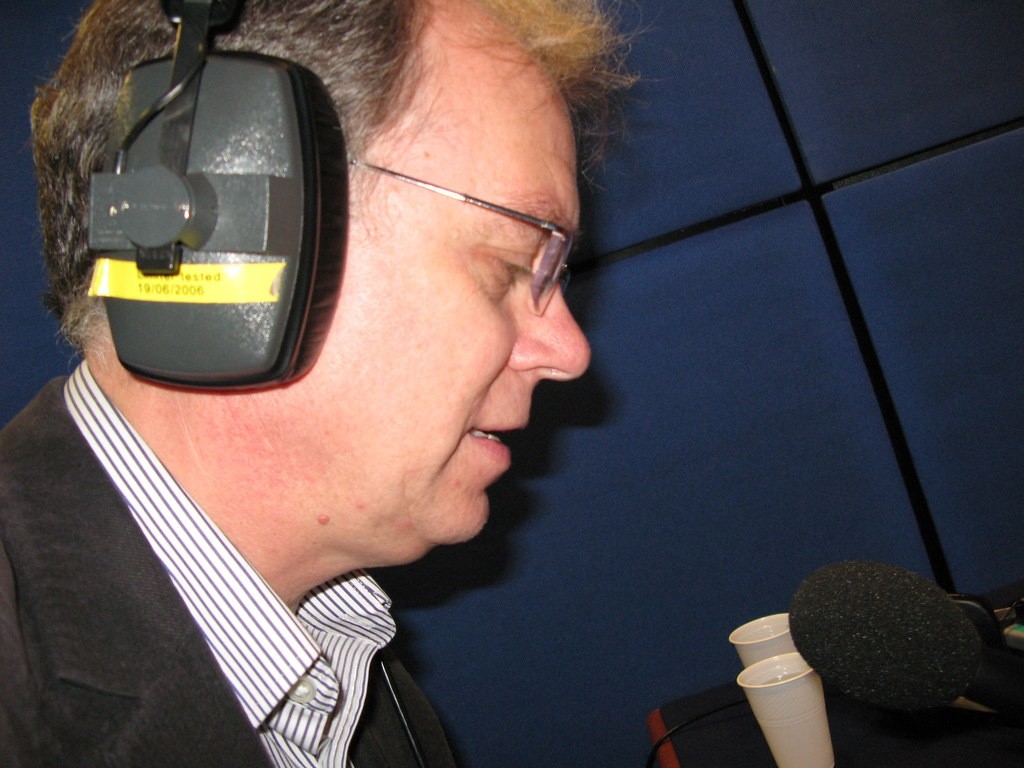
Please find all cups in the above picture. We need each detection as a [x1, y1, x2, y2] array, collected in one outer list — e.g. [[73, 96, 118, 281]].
[[736, 652, 835, 768], [729, 612, 797, 670]]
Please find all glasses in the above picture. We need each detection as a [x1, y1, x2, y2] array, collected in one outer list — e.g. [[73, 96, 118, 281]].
[[348, 156, 571, 315]]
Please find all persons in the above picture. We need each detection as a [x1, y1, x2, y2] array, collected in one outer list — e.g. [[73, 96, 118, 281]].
[[0, 0, 614, 768]]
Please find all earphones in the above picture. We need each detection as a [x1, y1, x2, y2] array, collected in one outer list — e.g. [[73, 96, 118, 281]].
[[90, 1, 321, 391]]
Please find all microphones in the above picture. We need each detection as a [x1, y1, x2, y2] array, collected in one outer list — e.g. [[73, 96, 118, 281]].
[[786, 559, 1023, 727]]
